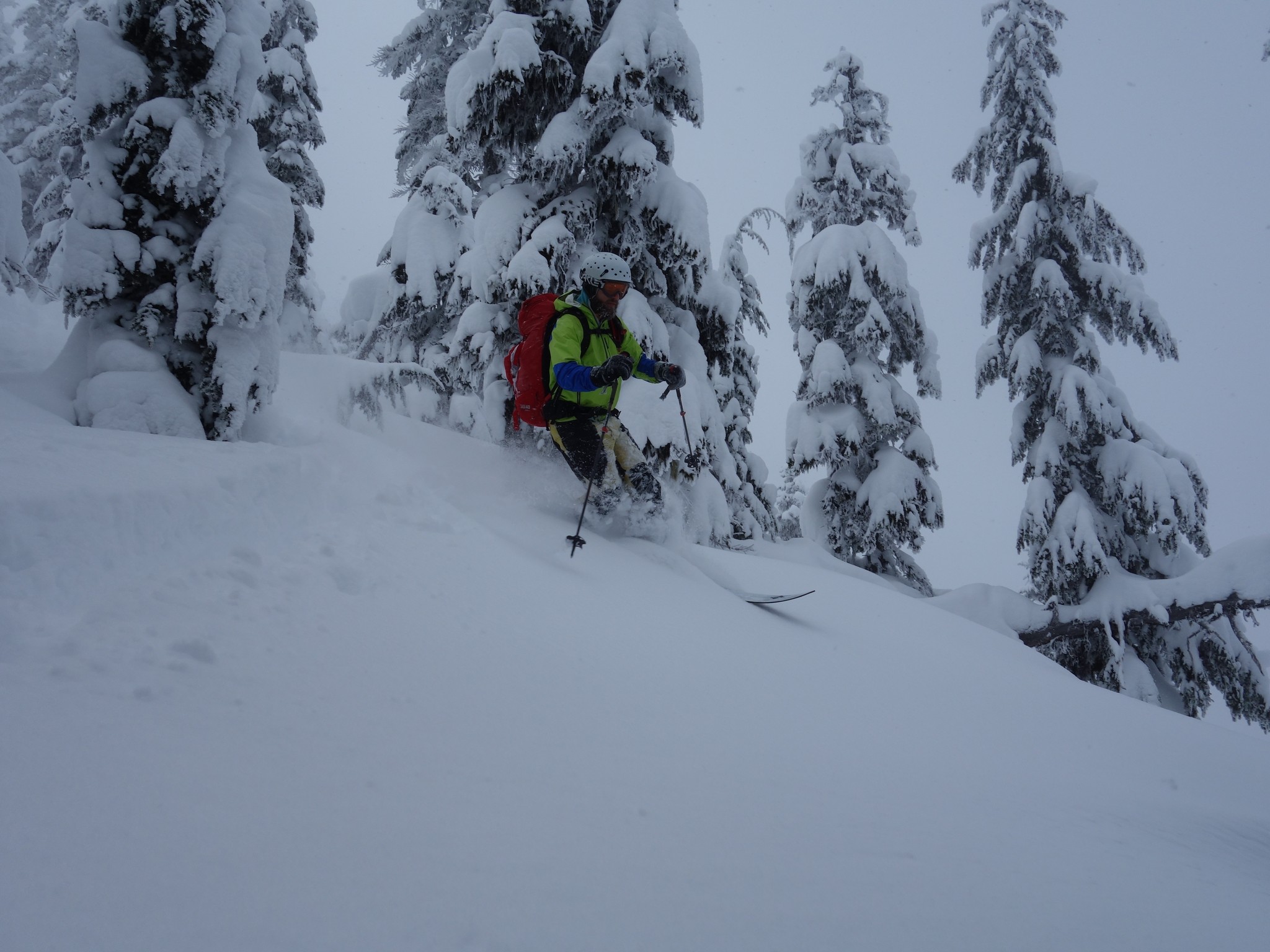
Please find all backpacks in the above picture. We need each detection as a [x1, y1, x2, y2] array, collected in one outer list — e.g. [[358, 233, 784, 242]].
[[503, 293, 623, 431]]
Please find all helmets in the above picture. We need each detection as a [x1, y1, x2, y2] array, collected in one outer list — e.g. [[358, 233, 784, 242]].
[[580, 252, 631, 288]]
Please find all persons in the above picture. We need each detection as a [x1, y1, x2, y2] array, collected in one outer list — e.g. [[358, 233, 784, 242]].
[[543, 252, 687, 542]]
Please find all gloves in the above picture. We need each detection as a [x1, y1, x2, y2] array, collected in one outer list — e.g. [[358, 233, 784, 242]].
[[655, 361, 687, 391], [590, 351, 633, 388]]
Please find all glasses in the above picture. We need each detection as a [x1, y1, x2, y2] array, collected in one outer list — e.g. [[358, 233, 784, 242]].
[[601, 280, 630, 300]]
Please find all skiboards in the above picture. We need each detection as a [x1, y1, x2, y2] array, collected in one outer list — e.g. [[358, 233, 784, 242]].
[[744, 590, 816, 603]]
